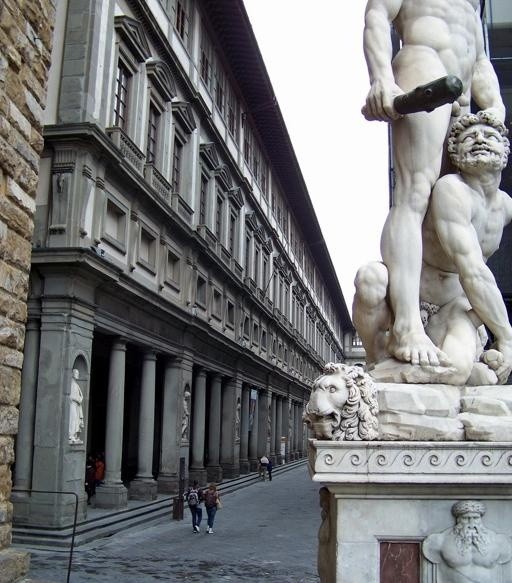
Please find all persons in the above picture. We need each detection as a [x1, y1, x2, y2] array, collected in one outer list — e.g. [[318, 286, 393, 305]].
[[83, 447, 105, 505], [362, 0, 507, 366], [199, 481, 223, 534], [421, 498, 511, 583], [256, 455, 267, 482], [266, 461, 273, 481], [350, 108, 512, 384], [181, 480, 205, 534]]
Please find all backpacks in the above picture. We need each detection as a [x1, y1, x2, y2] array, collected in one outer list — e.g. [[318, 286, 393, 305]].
[[188, 486, 200, 505], [205, 490, 216, 508]]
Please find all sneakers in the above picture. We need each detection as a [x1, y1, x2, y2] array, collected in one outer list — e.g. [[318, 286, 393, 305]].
[[194, 525, 214, 533]]
